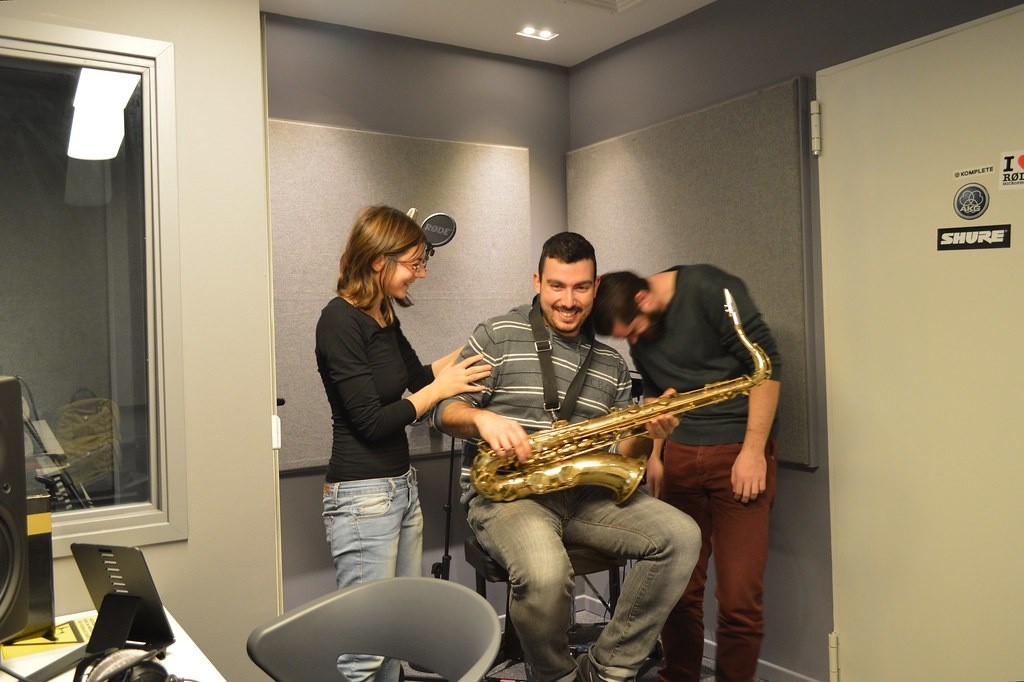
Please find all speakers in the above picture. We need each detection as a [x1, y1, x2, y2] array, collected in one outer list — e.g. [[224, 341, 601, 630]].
[[0, 376, 31, 641]]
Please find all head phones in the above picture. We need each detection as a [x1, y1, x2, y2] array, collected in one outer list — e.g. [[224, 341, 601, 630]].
[[73, 647, 182, 682]]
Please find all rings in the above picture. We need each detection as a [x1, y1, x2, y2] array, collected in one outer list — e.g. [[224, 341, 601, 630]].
[[467, 376, 471, 381]]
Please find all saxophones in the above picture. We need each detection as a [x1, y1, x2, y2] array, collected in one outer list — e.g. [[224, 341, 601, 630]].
[[470, 287, 772, 506]]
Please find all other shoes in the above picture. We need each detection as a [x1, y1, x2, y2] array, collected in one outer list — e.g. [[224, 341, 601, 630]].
[[576, 653, 604, 682]]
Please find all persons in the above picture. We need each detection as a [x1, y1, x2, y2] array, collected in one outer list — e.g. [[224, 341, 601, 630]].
[[316, 204, 492, 591], [431, 229, 703, 682], [587, 264, 781, 682]]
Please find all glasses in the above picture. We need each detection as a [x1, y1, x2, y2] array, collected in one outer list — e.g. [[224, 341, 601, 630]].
[[397, 259, 428, 272]]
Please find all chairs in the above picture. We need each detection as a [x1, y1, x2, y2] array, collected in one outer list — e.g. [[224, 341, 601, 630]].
[[54, 399, 122, 504], [247, 575, 502, 682], [460, 436, 629, 655]]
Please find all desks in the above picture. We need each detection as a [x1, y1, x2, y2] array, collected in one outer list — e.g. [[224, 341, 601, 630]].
[[0, 604, 227, 682]]
